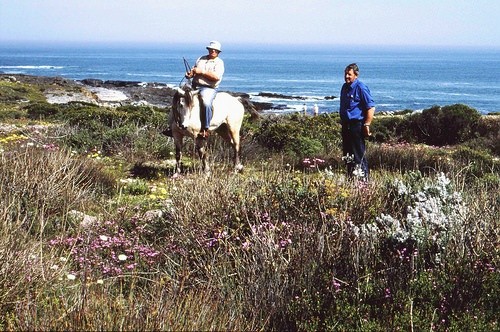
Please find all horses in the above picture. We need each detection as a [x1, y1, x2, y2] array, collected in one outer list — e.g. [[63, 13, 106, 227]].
[[169, 85, 259, 179]]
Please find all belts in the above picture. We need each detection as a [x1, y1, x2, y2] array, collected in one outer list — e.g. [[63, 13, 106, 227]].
[[193, 82, 212, 88]]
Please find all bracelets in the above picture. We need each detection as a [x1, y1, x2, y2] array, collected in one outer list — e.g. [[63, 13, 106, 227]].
[[201, 69, 206, 76], [363, 123, 370, 127]]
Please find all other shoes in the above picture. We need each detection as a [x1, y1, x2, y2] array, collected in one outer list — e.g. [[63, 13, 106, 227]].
[[198, 131, 208, 137], [162, 130, 173, 137]]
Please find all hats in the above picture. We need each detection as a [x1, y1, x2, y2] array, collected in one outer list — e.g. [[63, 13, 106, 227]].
[[206, 41, 222, 52]]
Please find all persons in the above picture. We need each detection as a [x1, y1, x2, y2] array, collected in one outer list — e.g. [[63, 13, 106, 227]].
[[162, 40, 225, 140], [339, 63, 376, 181], [302, 103, 307, 116], [313, 103, 319, 116]]
